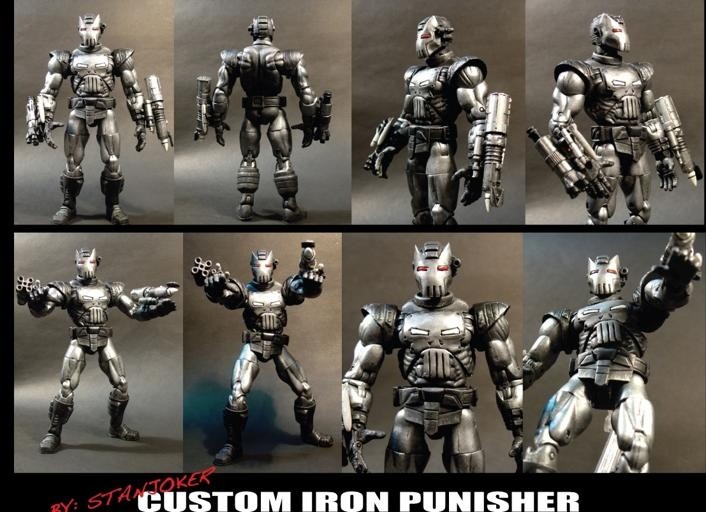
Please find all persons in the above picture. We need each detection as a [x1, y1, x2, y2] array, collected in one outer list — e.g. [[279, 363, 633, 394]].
[[189, 239, 335, 467], [193, 14, 333, 223], [523, 231, 703, 475], [338, 238, 524, 475], [15, 244, 179, 455], [525, 12, 703, 227], [359, 14, 512, 226], [26, 11, 172, 224]]
